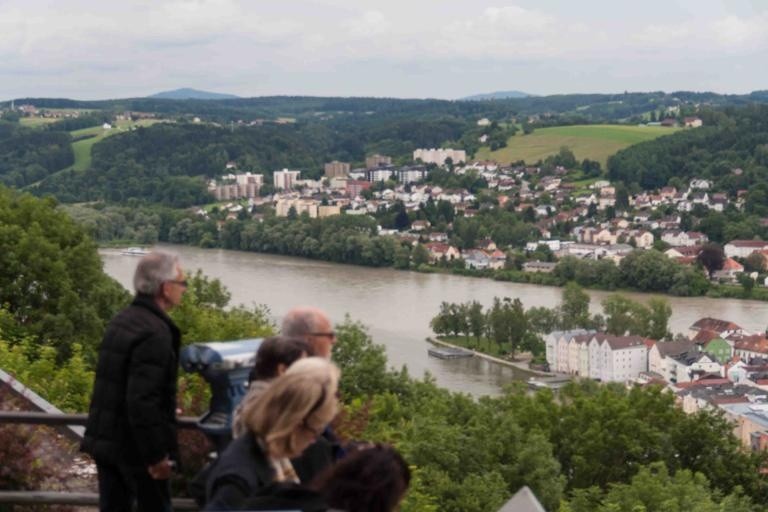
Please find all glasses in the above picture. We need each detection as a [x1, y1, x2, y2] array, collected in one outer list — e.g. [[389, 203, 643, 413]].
[[310, 333, 334, 339], [168, 280, 188, 287]]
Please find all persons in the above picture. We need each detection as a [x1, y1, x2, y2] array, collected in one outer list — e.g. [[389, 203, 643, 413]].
[[193, 306, 412, 512], [77, 250, 187, 510]]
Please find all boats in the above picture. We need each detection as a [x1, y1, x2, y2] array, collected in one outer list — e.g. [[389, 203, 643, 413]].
[[121, 246, 152, 259]]
